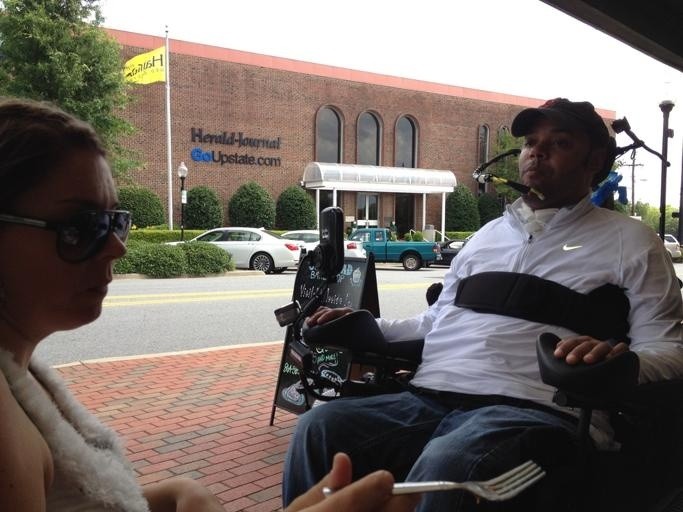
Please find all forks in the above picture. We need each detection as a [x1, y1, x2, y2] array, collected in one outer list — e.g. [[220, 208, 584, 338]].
[[322, 458, 545, 501]]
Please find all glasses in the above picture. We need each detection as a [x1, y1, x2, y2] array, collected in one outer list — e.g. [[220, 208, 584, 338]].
[[0, 209, 131, 264]]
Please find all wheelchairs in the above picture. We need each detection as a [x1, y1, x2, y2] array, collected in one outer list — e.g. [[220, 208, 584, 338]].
[[272, 273, 683, 512]]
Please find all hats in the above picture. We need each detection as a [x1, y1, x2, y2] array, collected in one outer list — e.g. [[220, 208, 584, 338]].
[[510, 98, 616, 186]]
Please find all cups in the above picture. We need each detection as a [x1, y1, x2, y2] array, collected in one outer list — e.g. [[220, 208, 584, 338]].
[[352, 272, 360, 283]]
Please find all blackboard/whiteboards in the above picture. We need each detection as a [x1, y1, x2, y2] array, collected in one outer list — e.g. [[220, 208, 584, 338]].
[[274, 252, 369, 415]]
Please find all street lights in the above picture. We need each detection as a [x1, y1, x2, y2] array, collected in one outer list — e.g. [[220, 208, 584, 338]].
[[657, 99, 675, 239], [177, 160, 189, 241]]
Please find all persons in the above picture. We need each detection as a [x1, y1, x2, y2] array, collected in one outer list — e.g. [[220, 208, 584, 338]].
[[282, 98, 682, 512], [0, 94, 422, 512]]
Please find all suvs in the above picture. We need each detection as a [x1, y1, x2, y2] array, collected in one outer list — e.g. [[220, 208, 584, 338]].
[[656, 231, 681, 260]]
